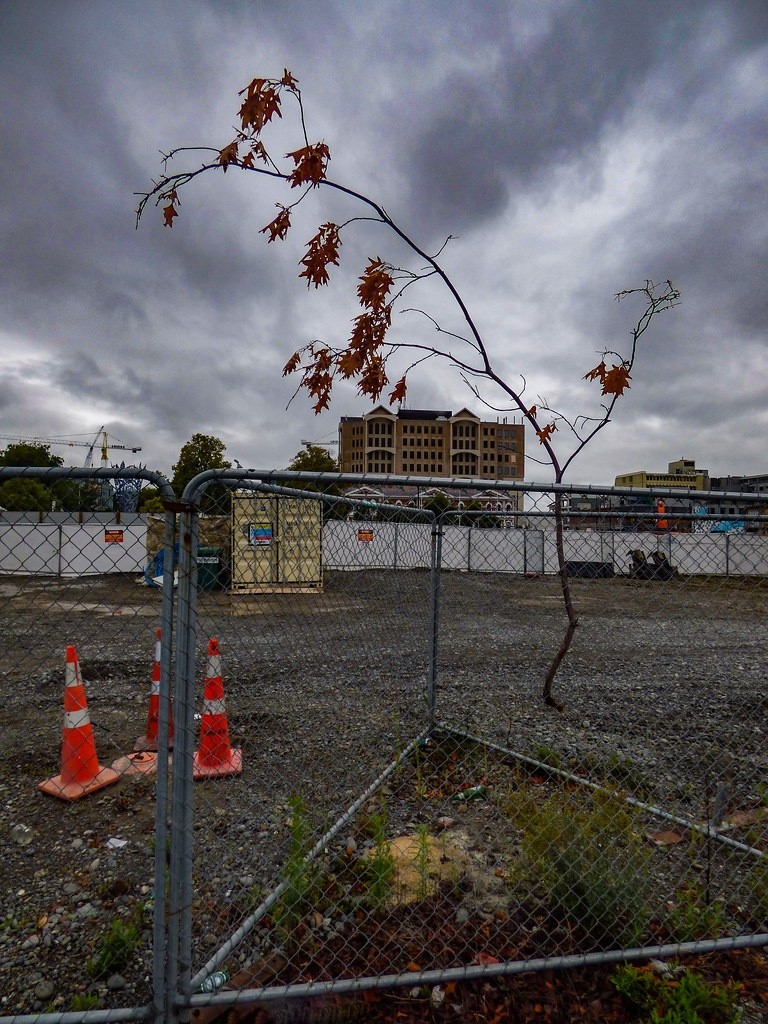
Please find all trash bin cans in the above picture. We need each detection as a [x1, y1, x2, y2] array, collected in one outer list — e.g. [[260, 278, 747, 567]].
[[197, 546, 221, 591]]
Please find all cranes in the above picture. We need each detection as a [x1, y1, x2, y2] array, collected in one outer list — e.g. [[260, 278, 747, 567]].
[[0, 432, 143, 468], [300, 429, 339, 446], [84, 426, 105, 467]]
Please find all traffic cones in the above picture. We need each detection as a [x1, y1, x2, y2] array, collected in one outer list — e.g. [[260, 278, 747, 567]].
[[37, 643, 121, 799], [132, 627, 176, 751], [189, 636, 243, 776]]
